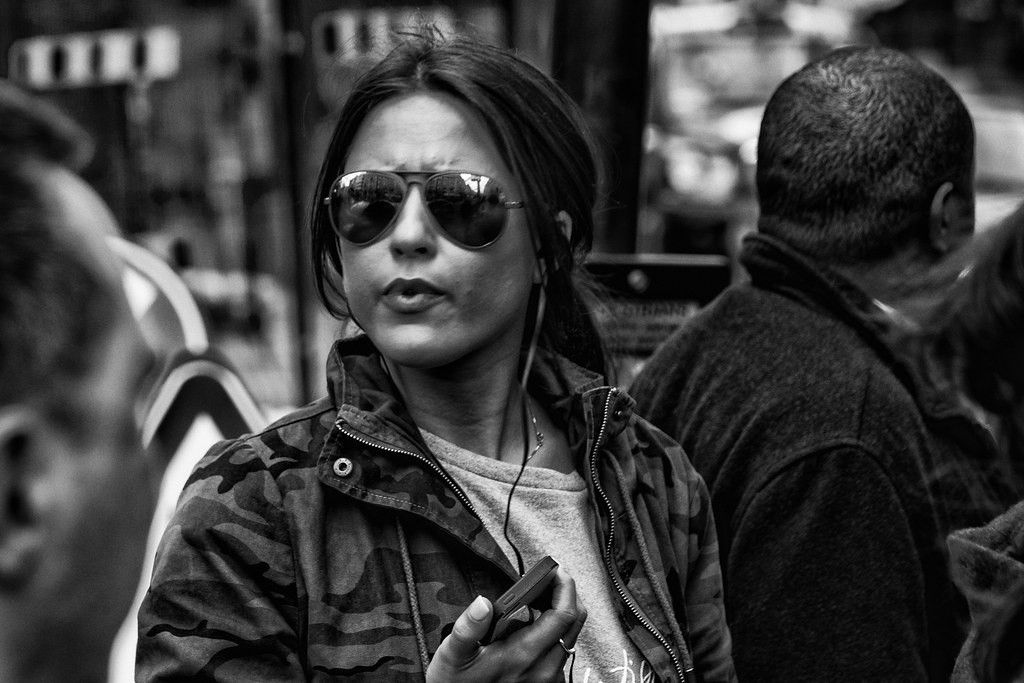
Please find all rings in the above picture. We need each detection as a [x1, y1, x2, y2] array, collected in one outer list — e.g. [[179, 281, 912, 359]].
[[559, 637, 575, 660]]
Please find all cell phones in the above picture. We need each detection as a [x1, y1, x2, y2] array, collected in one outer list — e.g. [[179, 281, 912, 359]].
[[478, 553, 560, 648]]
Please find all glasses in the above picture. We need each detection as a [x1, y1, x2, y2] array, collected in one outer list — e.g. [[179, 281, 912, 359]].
[[323, 170, 526, 250]]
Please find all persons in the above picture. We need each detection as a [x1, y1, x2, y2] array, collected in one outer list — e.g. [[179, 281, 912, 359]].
[[626, 44, 1024, 683], [134, 23, 742, 683], [0, 92, 159, 683]]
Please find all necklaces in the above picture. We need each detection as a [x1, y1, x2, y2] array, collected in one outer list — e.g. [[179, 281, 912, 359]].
[[522, 399, 547, 467]]
